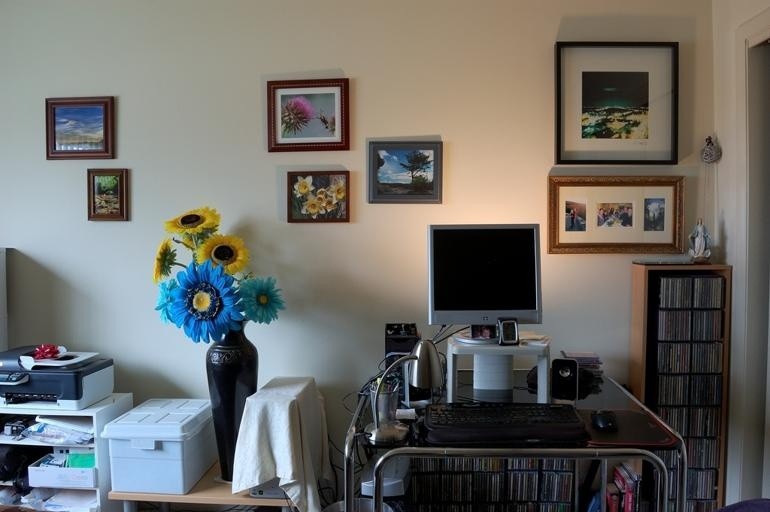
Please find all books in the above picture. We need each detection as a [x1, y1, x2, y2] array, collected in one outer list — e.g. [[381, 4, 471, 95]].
[[474, 456, 506, 472], [409, 474, 439, 502], [687, 468, 718, 500], [659, 310, 692, 341], [440, 473, 473, 503], [655, 450, 679, 468], [540, 456, 574, 472], [693, 309, 721, 341], [473, 472, 506, 503], [686, 501, 716, 511], [507, 457, 539, 471], [541, 503, 572, 511], [607, 462, 641, 512], [658, 374, 689, 405], [659, 406, 688, 437], [560, 350, 604, 377], [508, 471, 537, 501], [690, 406, 722, 437], [657, 342, 690, 374], [420, 503, 538, 512], [541, 471, 572, 502], [657, 473, 680, 511], [35, 416, 93, 435], [518, 331, 552, 348], [687, 438, 721, 469], [692, 342, 723, 373], [694, 278, 722, 308], [411, 457, 441, 473], [691, 374, 722, 406], [441, 456, 474, 472], [661, 278, 691, 309]]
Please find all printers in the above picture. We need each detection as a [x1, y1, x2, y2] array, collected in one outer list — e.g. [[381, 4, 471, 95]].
[[0, 346, 114, 410]]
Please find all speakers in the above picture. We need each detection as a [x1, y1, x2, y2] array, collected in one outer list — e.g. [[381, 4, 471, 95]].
[[550, 358, 578, 401], [496, 317, 520, 346]]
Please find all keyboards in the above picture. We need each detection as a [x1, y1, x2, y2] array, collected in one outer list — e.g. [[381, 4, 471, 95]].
[[423, 400, 585, 435]]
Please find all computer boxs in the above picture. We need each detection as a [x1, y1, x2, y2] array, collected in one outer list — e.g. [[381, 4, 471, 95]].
[[250, 478, 290, 499]]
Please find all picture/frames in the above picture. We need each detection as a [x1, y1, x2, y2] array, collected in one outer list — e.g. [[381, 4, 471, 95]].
[[45, 97, 112, 159], [552, 40, 681, 166], [367, 141, 442, 205], [86, 167, 128, 220], [265, 77, 348, 152], [546, 175, 684, 256], [287, 172, 350, 224]]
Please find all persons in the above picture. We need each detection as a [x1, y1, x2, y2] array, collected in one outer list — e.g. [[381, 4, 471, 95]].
[[689, 218, 712, 257]]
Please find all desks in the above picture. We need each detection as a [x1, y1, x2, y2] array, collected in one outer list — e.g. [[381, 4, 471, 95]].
[[108, 465, 298, 512], [343, 371, 688, 512]]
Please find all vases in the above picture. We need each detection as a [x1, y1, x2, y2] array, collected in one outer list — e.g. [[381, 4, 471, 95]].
[[205, 321, 260, 483]]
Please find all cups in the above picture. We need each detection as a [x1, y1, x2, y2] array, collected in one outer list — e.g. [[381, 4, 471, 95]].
[[369, 387, 399, 424]]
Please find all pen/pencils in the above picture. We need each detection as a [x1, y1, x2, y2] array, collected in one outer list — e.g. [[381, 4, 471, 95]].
[[388, 379, 399, 399]]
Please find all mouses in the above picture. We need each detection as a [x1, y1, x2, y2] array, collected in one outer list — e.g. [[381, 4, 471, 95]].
[[592, 413, 619, 435]]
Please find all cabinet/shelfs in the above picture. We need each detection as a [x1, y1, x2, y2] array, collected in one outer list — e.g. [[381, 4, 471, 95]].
[[630, 263, 732, 511], [409, 456, 577, 512], [1, 392, 134, 512]]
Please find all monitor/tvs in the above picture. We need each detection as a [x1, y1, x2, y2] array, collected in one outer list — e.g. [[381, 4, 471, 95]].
[[427, 223, 542, 346]]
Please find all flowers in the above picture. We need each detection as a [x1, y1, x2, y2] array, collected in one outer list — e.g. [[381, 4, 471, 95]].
[[148, 206, 286, 345]]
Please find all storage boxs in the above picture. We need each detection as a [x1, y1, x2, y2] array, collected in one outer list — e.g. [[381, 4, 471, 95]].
[[99, 398, 218, 495]]
